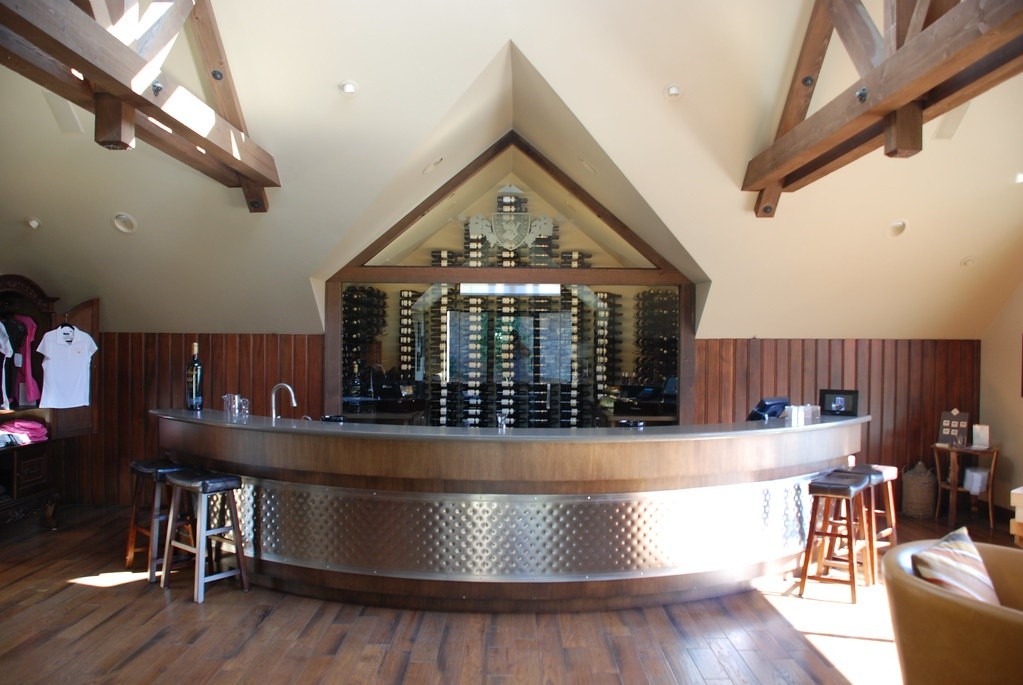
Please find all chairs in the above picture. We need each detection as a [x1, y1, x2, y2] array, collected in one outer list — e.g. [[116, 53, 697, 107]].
[[882, 539, 1023, 685]]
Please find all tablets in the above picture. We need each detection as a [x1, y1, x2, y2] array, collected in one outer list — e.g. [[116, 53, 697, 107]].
[[820, 389, 859, 417]]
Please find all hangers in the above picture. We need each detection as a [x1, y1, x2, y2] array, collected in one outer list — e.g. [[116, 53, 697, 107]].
[[61, 312, 75, 342]]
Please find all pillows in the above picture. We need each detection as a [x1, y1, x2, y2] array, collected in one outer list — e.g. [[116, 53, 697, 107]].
[[912, 527, 1001, 608]]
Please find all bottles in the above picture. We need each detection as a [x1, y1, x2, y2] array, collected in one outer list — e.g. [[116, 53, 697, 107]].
[[400, 284, 631, 427], [340, 287, 388, 414], [561, 251, 592, 260], [463, 197, 560, 268], [186, 342, 203, 411], [431, 260, 462, 267], [633, 289, 680, 396], [431, 251, 463, 259], [785, 403, 821, 422], [561, 261, 591, 267], [222, 393, 250, 414]]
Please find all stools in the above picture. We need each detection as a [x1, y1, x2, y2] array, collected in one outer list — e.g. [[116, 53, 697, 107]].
[[823, 465, 898, 585], [798, 471, 872, 603], [160, 468, 249, 604], [125, 458, 197, 582]]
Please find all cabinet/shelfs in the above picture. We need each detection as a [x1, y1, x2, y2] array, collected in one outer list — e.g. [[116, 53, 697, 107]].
[[0, 273, 100, 531], [931, 444, 999, 529]]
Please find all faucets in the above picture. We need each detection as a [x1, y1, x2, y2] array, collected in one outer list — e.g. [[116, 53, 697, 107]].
[[270, 382, 298, 418]]
